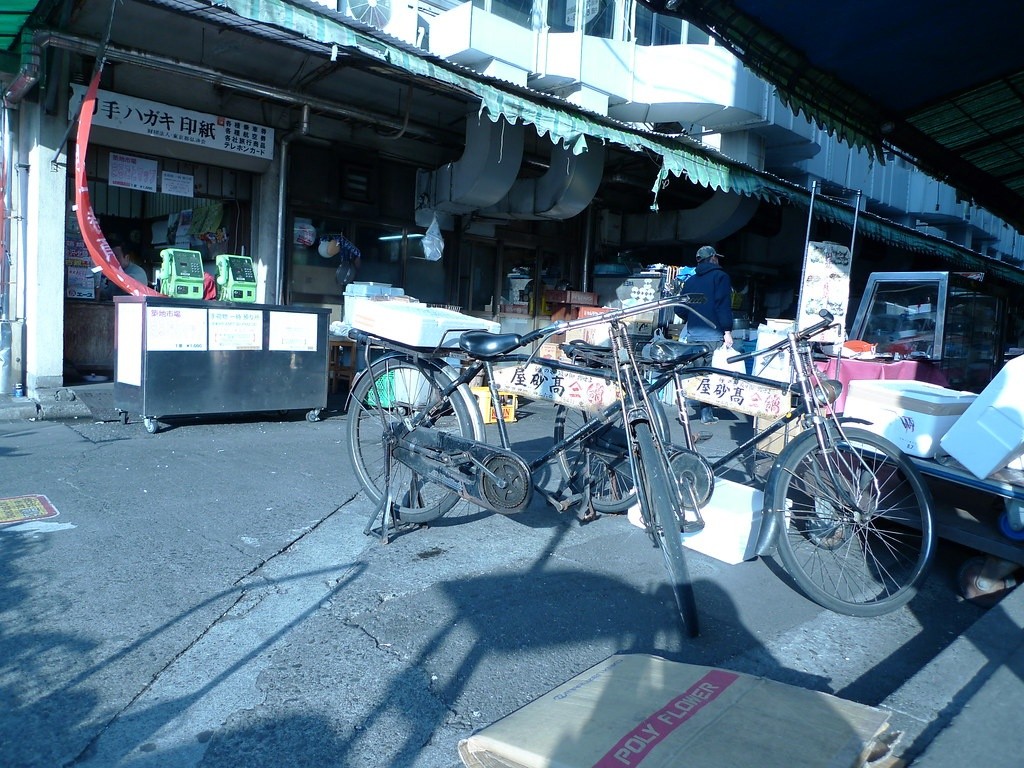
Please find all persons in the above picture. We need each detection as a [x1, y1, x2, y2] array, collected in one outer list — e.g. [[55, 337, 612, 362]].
[[674, 246, 733, 424], [97, 243, 148, 301]]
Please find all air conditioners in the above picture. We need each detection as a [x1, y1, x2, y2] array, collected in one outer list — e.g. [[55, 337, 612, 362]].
[[336, 0, 419, 47]]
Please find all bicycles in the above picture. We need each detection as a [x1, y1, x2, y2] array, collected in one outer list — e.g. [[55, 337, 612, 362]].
[[554, 308, 939, 616], [337, 293, 718, 639]]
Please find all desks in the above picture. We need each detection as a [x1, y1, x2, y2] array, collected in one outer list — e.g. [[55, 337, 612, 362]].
[[809, 357, 951, 418], [328, 340, 357, 390], [63, 299, 114, 371]]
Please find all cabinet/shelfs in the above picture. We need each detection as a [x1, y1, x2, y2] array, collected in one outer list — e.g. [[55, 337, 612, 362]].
[[112, 295, 332, 434], [851, 269, 1007, 366]]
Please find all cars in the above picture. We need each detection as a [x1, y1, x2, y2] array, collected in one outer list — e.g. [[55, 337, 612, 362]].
[[865, 313, 935, 354]]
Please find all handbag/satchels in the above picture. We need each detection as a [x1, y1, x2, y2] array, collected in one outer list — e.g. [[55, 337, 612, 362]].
[[712, 343, 747, 375], [678, 321, 688, 343]]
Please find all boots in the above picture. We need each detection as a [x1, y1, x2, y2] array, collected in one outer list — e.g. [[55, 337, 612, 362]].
[[699, 401, 719, 425]]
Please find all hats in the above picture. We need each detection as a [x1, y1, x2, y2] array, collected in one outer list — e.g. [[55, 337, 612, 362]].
[[696, 245, 724, 262]]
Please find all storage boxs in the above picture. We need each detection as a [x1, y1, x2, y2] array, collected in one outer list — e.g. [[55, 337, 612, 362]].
[[749, 331, 757, 341], [939, 354, 1024, 482], [732, 330, 749, 340], [342, 281, 654, 361], [470, 386, 519, 423], [593, 264, 628, 275], [765, 318, 795, 332], [840, 380, 979, 458]]
